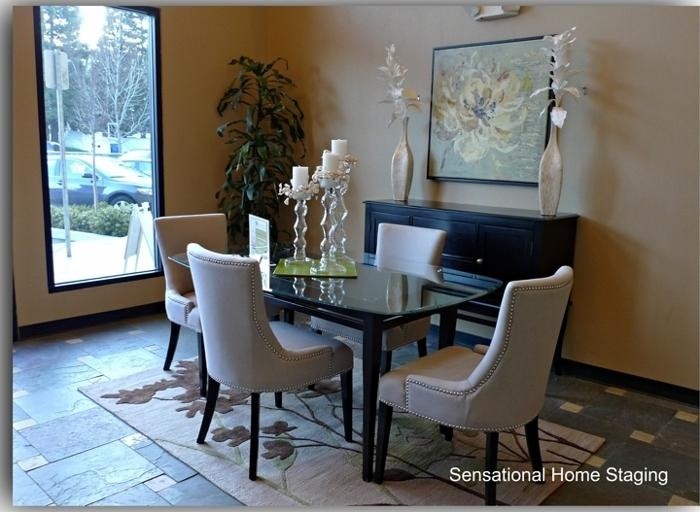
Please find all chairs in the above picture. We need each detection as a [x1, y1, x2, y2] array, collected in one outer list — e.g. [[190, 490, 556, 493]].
[[188, 243, 354, 480], [376, 265, 573, 505], [155, 212, 227, 397]]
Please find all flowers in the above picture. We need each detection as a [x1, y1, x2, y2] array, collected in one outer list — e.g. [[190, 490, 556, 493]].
[[378, 43, 421, 127], [529, 28, 582, 129], [435, 54, 533, 171]]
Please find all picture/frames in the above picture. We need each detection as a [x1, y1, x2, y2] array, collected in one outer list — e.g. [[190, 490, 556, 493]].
[[427, 34, 555, 187]]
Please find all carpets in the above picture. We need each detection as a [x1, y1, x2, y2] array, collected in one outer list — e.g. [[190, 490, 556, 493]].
[[78, 351, 606, 506]]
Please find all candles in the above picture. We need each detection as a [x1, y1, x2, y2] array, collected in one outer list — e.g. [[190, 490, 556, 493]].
[[323, 152, 340, 171], [331, 139, 348, 161], [292, 166, 309, 190]]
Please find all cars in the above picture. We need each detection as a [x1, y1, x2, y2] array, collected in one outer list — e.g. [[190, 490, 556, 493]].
[[48, 156, 153, 215], [47, 142, 62, 153], [119, 151, 152, 176]]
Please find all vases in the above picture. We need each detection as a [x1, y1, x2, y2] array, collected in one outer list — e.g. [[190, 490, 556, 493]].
[[390, 117, 413, 202], [540, 115, 563, 217]]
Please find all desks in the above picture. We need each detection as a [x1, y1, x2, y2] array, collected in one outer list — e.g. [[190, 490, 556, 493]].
[[362, 198, 580, 376]]
